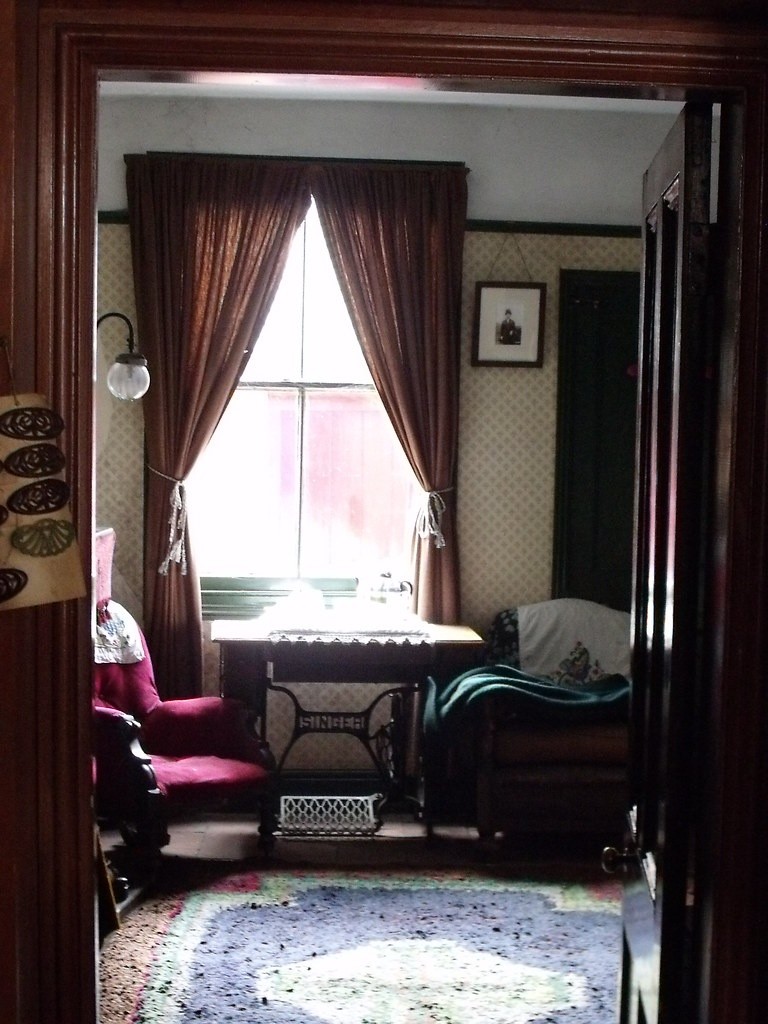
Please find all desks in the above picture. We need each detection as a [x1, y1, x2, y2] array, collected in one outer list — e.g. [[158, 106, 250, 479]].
[[207, 617, 486, 838]]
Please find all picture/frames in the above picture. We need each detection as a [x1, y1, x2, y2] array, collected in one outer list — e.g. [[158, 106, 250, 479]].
[[471, 281, 547, 368]]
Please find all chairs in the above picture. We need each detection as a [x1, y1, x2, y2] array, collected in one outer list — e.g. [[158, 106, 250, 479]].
[[478, 597, 630, 866], [92, 601, 280, 894]]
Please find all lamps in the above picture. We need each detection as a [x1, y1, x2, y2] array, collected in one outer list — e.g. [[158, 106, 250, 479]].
[[98, 312, 151, 401]]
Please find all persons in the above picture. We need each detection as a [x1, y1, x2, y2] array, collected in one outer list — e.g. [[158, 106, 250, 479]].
[[499, 308, 516, 344]]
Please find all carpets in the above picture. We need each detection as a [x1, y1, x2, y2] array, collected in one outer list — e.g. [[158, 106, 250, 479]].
[[100, 869, 623, 1024]]
